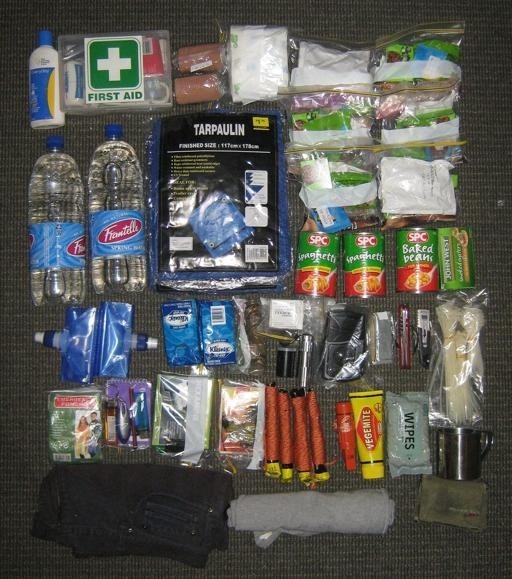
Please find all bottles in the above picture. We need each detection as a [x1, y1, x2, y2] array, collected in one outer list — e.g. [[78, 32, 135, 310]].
[[86, 125, 147, 294], [26, 136, 87, 305], [29, 28, 67, 127]]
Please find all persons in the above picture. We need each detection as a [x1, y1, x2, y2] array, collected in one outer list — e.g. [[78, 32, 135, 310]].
[[87, 411, 103, 458], [75, 415, 93, 459]]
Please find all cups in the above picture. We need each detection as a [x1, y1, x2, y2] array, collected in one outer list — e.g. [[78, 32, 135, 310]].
[[436, 426, 495, 481]]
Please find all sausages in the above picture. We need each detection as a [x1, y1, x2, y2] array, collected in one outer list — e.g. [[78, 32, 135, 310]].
[[178, 42, 227, 72], [175, 74, 224, 104]]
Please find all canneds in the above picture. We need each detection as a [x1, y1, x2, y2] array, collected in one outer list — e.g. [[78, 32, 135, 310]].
[[295, 230, 340, 297], [395, 228, 439, 292], [342, 230, 386, 299]]
